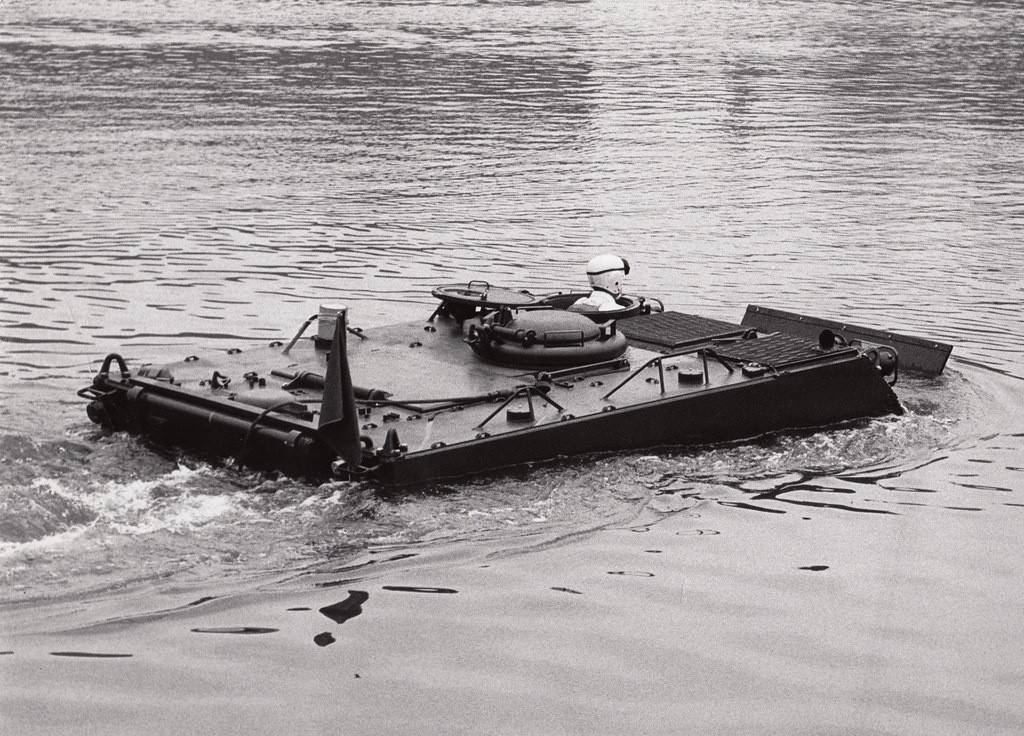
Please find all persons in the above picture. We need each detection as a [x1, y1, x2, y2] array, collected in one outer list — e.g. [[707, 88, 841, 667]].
[[573, 255, 631, 311]]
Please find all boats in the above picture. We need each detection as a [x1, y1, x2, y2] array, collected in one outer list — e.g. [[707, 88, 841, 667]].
[[72, 252, 956, 499]]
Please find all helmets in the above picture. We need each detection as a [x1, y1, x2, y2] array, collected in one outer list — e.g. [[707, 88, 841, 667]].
[[586, 255, 626, 295]]
